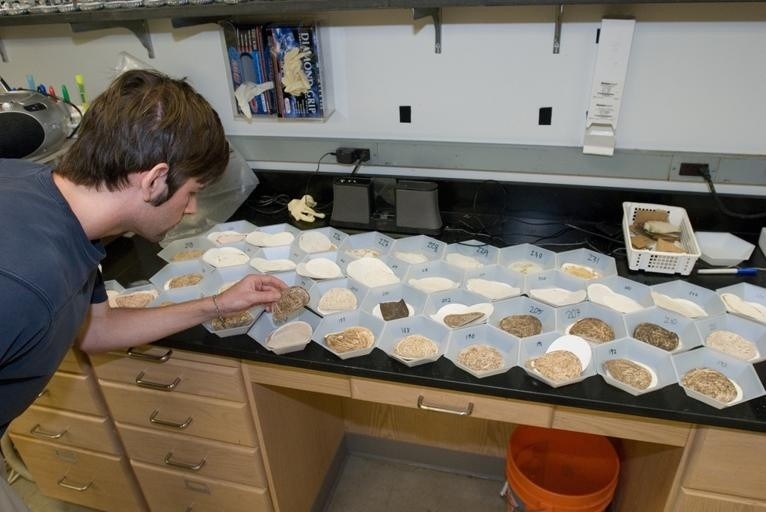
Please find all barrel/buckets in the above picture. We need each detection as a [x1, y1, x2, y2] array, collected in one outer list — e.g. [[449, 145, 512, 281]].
[[501, 425, 620, 512]]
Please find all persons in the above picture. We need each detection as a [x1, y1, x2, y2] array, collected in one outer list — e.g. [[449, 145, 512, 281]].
[[0, 68, 292, 440]]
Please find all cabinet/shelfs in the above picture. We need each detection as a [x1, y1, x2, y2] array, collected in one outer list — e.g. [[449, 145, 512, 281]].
[[7, 338, 150, 510], [667, 424, 765, 510], [348, 378, 554, 430], [84, 346, 276, 510]]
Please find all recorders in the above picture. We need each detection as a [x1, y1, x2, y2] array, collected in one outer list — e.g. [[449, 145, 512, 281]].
[[0, 88, 70, 162]]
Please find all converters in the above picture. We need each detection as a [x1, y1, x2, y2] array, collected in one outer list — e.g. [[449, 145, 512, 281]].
[[336, 147, 357, 164]]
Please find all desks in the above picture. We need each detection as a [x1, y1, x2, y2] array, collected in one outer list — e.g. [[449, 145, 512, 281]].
[[7, 189, 765, 510]]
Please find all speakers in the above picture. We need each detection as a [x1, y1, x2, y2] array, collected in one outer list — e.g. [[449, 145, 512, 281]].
[[330, 179, 374, 223], [393, 179, 443, 229]]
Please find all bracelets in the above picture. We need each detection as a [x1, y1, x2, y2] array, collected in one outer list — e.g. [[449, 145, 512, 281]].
[[211, 295, 223, 323]]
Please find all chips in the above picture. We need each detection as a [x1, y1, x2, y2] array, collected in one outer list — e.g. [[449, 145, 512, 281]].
[[115, 231, 763, 404], [629, 210, 689, 255]]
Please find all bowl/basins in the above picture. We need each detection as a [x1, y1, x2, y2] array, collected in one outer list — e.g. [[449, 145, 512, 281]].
[[104, 220, 766, 410]]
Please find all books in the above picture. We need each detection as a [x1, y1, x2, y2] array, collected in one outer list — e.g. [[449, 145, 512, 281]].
[[224, 20, 329, 120]]
